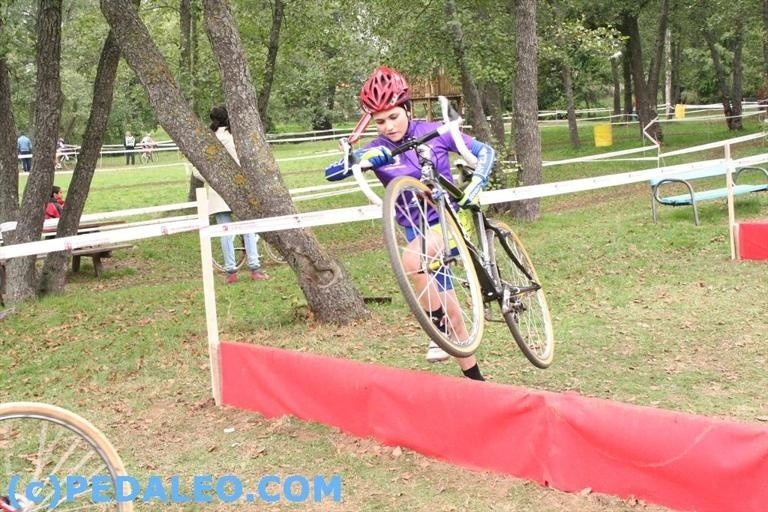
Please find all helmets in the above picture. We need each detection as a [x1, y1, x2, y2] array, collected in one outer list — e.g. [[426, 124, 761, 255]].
[[360, 66, 411, 115]]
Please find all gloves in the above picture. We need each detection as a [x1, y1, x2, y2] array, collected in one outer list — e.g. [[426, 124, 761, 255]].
[[456, 174, 487, 209], [354, 144, 396, 168]]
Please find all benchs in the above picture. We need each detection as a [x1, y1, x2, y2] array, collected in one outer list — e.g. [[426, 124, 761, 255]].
[[651, 166, 767, 224], [36, 244, 132, 280]]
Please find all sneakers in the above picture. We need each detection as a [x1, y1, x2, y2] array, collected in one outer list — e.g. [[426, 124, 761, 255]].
[[251, 270, 271, 281], [224, 273, 238, 284], [425, 330, 451, 363]]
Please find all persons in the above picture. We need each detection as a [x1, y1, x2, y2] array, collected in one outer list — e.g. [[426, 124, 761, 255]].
[[191, 106, 269, 283], [17, 131, 32, 171], [124, 130, 136, 164], [55, 137, 65, 169], [325, 65, 495, 380], [141, 133, 154, 158], [43, 186, 65, 219]]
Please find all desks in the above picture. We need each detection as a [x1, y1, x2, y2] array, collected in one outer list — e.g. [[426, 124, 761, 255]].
[[42, 220, 126, 278]]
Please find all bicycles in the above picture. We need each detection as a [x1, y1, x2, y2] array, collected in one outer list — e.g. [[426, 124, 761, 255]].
[[55, 138, 77, 171], [338, 95, 556, 370], [211, 233, 288, 272], [140, 144, 159, 167], [0, 400, 135, 512]]
